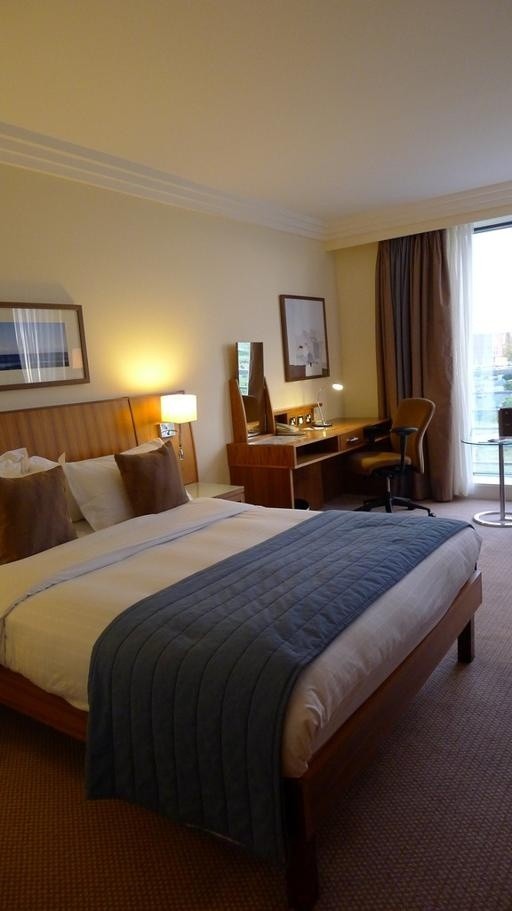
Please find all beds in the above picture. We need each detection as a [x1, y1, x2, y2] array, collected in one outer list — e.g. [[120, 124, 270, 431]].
[[0, 393, 484, 901]]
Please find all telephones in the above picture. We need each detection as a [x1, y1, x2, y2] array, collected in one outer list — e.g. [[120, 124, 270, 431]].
[[275, 423, 299, 433]]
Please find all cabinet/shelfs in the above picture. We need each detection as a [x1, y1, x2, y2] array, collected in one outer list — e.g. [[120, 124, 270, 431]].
[[186, 482, 243, 502], [225, 410, 391, 510]]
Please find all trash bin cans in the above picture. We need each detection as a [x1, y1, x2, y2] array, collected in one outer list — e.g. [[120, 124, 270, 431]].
[[294, 498, 311, 510]]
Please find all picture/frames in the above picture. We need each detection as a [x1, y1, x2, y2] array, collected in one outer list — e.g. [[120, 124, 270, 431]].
[[282, 294, 333, 382], [0, 300, 90, 388]]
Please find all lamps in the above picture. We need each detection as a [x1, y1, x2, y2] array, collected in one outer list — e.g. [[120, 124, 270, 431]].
[[309, 380, 345, 432], [159, 391, 202, 492]]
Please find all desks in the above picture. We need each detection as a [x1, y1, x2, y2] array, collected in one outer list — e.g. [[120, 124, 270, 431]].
[[461, 432, 511, 529]]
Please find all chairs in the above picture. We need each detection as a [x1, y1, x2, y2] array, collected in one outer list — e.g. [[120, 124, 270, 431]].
[[358, 398, 438, 517]]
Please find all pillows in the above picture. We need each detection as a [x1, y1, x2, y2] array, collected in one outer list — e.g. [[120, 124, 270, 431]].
[[110, 445, 188, 513], [0, 467, 76, 561], [54, 440, 170, 531]]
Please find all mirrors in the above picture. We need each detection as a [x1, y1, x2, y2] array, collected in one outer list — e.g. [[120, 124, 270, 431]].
[[232, 339, 273, 434]]
